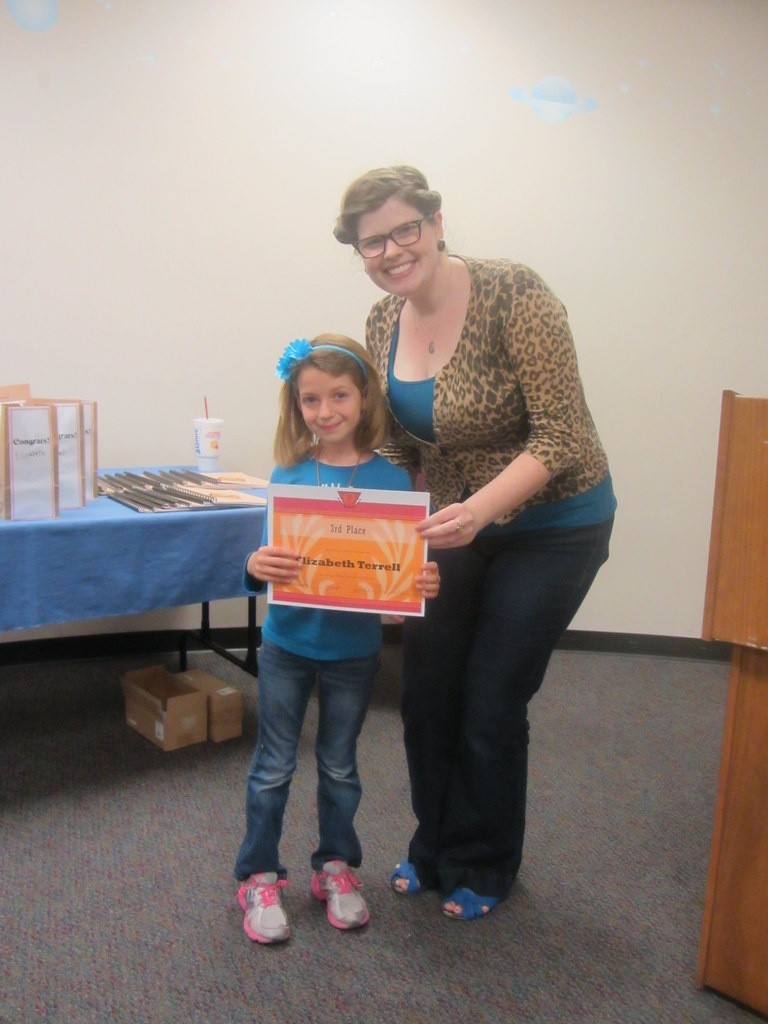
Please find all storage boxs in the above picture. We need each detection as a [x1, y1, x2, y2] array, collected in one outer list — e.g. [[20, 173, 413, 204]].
[[173, 667, 244, 746], [119, 664, 212, 752]]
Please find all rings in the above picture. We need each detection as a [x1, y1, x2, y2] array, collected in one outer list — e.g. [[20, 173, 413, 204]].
[[453, 518, 465, 533]]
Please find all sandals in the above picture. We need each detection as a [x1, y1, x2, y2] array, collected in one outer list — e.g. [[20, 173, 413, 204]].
[[390, 858, 424, 895], [440, 885, 500, 921]]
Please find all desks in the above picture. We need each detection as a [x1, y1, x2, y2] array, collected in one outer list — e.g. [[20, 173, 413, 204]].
[[1, 465, 271, 679]]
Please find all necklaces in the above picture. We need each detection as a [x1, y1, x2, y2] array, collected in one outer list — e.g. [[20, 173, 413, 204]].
[[408, 255, 454, 356], [315, 437, 362, 488]]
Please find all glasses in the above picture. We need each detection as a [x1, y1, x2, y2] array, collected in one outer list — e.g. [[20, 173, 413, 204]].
[[350, 205, 440, 260]]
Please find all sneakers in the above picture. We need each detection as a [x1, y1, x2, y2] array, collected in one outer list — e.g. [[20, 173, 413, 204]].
[[310, 858, 371, 931], [236, 870, 292, 947]]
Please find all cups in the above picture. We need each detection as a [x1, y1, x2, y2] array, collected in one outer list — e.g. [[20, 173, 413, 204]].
[[193, 417, 224, 472]]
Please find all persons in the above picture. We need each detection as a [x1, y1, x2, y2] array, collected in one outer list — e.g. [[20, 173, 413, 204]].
[[329, 165, 617, 920], [232, 332, 441, 946]]
